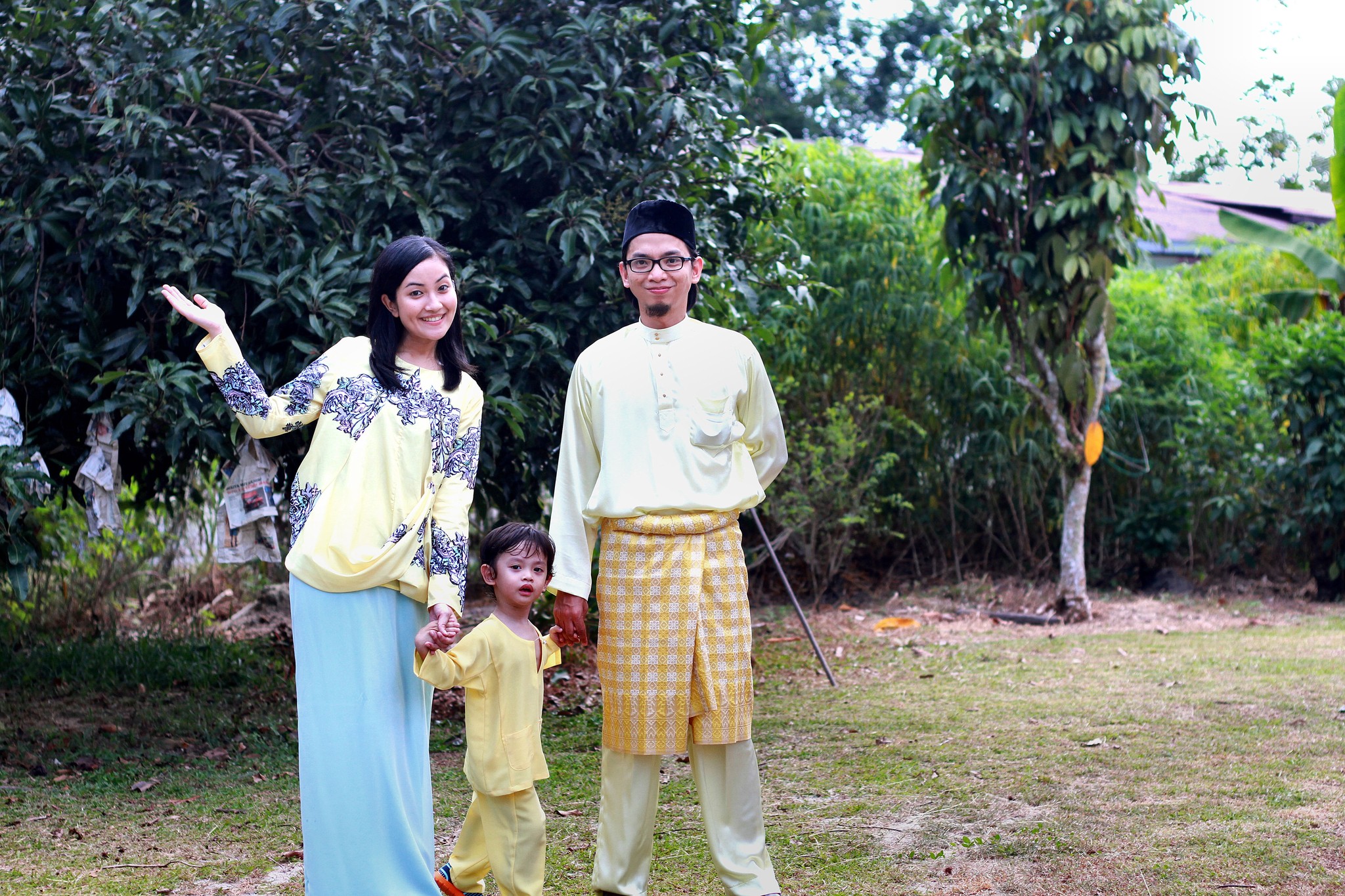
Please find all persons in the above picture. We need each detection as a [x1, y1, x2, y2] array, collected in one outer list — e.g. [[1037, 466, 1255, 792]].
[[551, 195, 787, 896], [160, 237, 483, 896], [413, 524, 581, 896]]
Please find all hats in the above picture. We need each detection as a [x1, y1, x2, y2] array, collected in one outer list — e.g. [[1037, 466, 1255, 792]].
[[621, 199, 699, 313]]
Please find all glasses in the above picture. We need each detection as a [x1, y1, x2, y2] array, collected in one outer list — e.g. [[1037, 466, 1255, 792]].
[[626, 257, 696, 273]]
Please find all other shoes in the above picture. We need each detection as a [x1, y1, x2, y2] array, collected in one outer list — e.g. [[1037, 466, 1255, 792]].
[[434, 865, 483, 896]]
